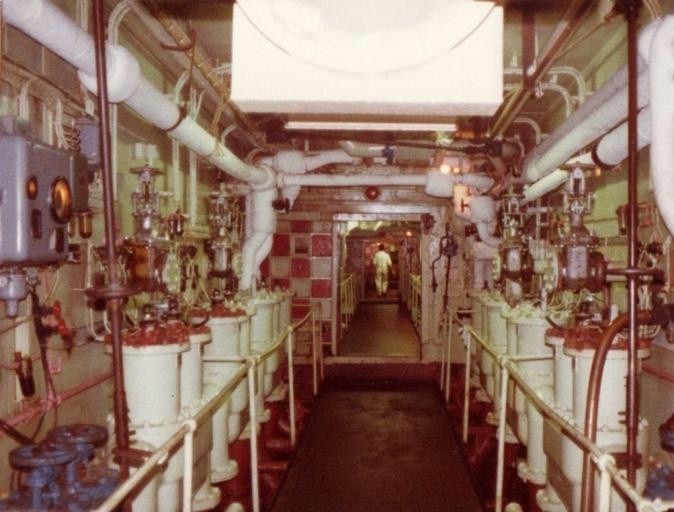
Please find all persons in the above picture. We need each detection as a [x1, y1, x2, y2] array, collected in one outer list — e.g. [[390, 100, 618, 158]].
[[370, 243, 396, 298], [361, 247, 372, 281], [388, 244, 398, 281]]
